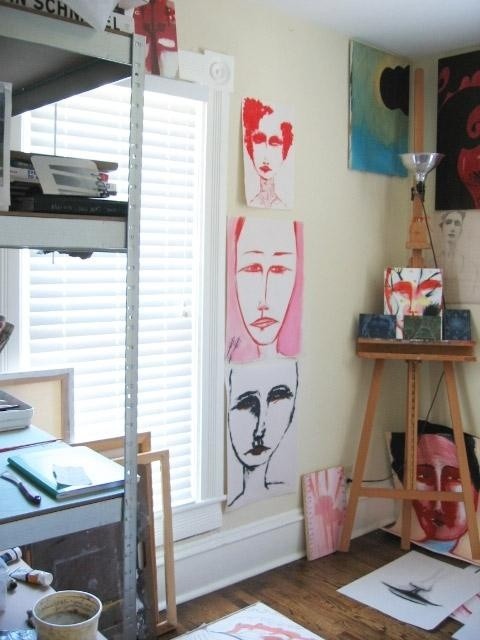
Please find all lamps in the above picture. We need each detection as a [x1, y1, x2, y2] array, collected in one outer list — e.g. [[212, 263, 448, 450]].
[[400, 150, 443, 189]]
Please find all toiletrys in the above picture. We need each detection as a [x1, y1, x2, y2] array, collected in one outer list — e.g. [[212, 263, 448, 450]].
[[7, 567, 55, 587]]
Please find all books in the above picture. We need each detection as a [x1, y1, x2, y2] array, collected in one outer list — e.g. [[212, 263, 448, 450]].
[[7, 446, 141, 499]]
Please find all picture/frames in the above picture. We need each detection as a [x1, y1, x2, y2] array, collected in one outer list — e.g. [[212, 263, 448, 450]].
[[72, 445, 182, 639], [23, 429, 159, 640], [0, 367, 80, 450]]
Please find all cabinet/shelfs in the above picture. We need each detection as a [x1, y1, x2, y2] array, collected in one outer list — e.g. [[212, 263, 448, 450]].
[[0, 0, 150, 640]]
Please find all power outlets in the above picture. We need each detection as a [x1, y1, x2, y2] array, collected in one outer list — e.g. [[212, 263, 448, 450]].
[[344, 464, 353, 489]]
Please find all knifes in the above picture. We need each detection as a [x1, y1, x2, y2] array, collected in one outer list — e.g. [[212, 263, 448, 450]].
[[0, 468, 41, 504]]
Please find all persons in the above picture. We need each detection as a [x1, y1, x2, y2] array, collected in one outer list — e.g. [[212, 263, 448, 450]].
[[430, 210, 466, 270], [225, 360, 298, 511], [243, 98, 293, 209], [392, 419, 480, 553], [234, 217, 297, 359]]
